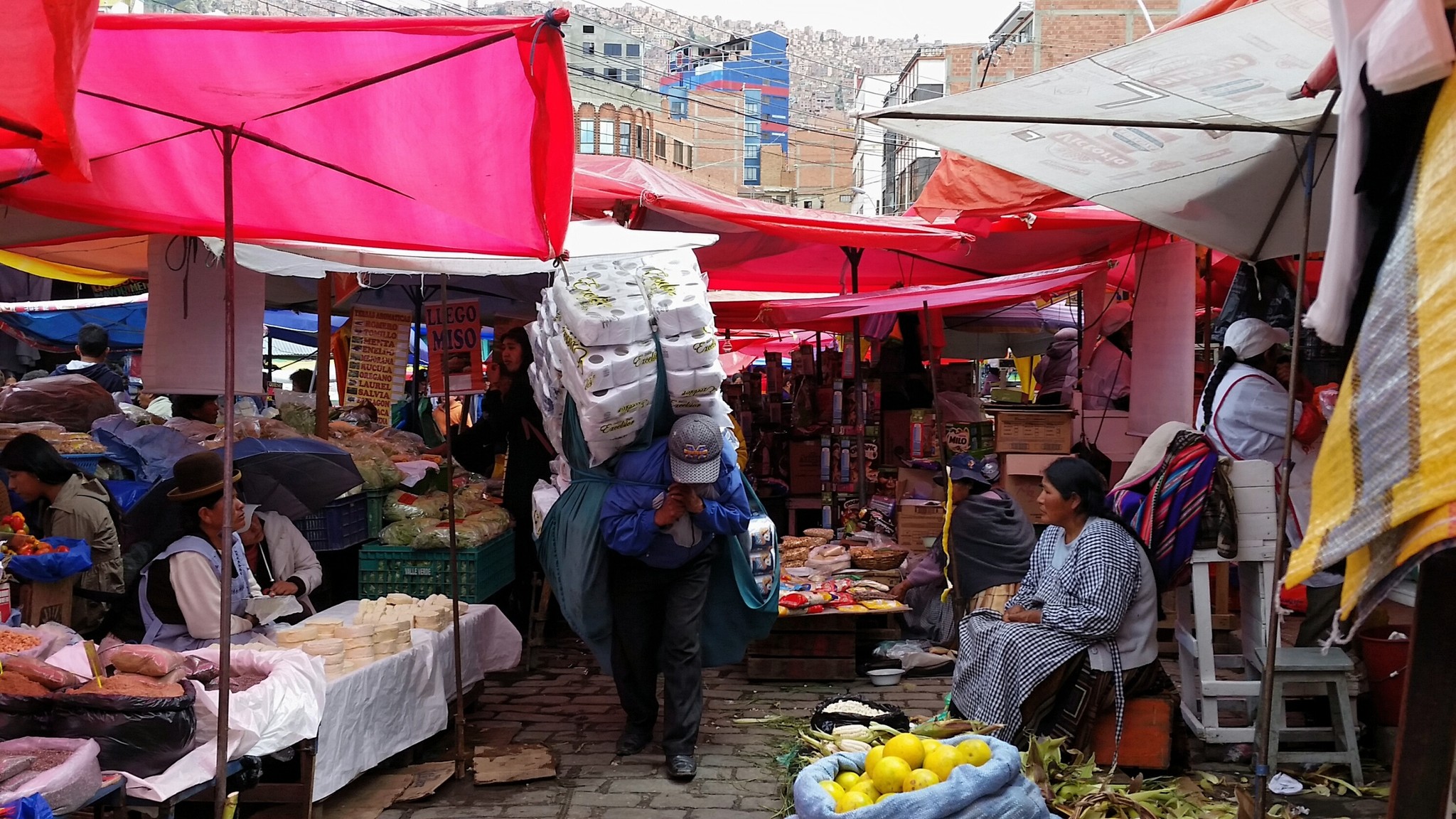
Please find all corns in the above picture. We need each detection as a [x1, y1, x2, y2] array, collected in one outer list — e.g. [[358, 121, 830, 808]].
[[806, 725, 879, 763]]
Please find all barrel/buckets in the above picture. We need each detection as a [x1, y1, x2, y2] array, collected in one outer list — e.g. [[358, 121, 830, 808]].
[[1357, 624, 1413, 727]]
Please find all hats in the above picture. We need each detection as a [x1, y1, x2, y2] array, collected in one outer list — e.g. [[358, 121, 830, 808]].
[[932, 453, 992, 494], [1099, 302, 1134, 336], [1053, 327, 1078, 341], [236, 503, 262, 533], [166, 450, 242, 502], [669, 414, 723, 484], [1224, 318, 1290, 361]]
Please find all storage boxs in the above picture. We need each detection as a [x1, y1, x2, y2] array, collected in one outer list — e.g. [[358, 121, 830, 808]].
[[893, 500, 950, 550], [995, 412, 1071, 456], [364, 487, 388, 539], [359, 527, 518, 603], [3, 453, 102, 522], [791, 439, 834, 494], [9, 569, 73, 629], [897, 468, 950, 502], [292, 492, 370, 553], [998, 452, 1077, 525], [946, 419, 996, 462]]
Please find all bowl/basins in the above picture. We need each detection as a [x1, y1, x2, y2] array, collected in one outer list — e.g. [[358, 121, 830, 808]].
[[865, 669, 905, 686]]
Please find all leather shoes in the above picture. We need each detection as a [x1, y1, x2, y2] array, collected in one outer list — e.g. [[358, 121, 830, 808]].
[[616, 730, 654, 755], [664, 755, 698, 777]]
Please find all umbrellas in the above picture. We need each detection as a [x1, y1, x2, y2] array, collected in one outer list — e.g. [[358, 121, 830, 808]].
[[1, 4, 581, 819], [0, 148, 1131, 506], [851, 1, 1355, 819]]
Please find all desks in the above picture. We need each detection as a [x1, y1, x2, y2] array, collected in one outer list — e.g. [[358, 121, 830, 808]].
[[158, 600, 496, 819]]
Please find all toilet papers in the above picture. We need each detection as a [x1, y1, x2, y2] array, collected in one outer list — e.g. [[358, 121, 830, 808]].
[[530, 247, 740, 547], [735, 511, 776, 600]]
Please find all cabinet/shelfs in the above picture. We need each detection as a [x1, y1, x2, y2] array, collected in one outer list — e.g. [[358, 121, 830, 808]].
[[1167, 451, 1283, 752]]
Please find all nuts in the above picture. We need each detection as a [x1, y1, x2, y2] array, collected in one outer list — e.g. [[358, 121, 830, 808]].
[[0, 630, 41, 654]]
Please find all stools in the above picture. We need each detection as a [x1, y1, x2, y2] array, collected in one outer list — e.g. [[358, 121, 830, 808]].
[[1256, 643, 1365, 787]]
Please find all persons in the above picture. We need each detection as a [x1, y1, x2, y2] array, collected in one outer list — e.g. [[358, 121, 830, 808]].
[[0, 434, 125, 641], [601, 412, 753, 782], [880, 458, 1038, 654], [416, 324, 562, 622], [1196, 318, 1323, 641], [137, 452, 309, 784], [942, 456, 1159, 763], [236, 503, 322, 628]]
